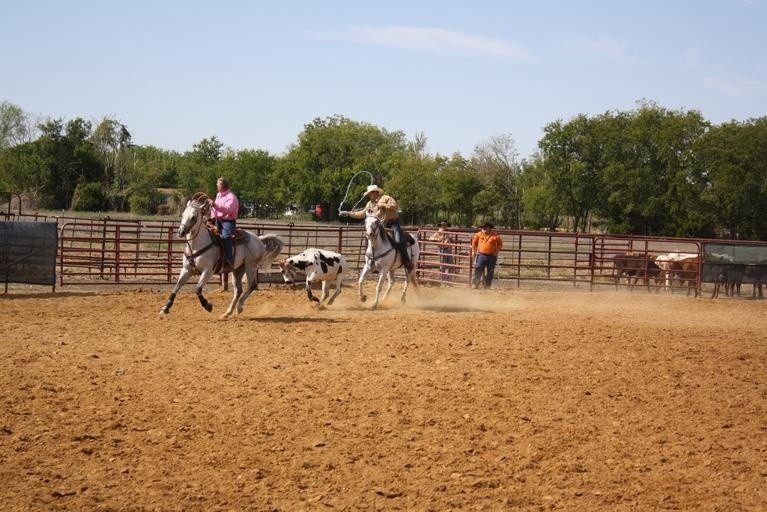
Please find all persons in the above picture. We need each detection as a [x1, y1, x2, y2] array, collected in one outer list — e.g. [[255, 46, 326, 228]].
[[340, 185, 414, 273], [429, 222, 454, 281], [206, 178, 239, 271], [240, 203, 298, 217], [471, 222, 503, 289], [311, 204, 324, 222]]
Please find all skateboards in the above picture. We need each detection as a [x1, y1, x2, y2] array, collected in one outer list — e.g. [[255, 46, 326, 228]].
[[280, 247, 347, 306], [612, 250, 767, 299]]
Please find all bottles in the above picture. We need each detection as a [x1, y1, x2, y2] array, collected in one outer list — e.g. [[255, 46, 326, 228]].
[[362, 184, 384, 197], [437, 221, 450, 227], [477, 223, 496, 229]]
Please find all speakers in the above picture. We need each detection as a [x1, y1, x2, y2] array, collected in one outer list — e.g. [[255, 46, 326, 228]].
[[224, 260, 233, 272], [404, 259, 413, 270]]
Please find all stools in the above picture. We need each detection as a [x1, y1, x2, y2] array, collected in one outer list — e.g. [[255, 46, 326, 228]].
[[480, 253, 493, 256]]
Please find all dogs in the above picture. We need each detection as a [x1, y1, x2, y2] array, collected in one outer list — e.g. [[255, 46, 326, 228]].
[[158, 199, 283, 318], [357, 208, 421, 310]]
